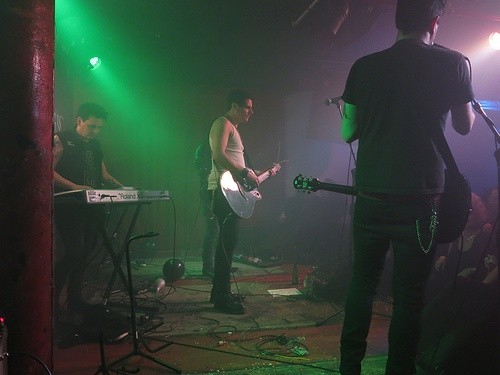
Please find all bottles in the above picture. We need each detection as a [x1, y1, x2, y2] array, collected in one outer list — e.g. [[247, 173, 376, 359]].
[[292, 264, 298, 285]]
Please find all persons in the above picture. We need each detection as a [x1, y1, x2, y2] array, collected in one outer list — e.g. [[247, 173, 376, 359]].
[[448, 187, 500, 283], [52, 103, 124, 303], [342, 0, 475, 375], [208, 89, 277, 315], [197, 141, 238, 277]]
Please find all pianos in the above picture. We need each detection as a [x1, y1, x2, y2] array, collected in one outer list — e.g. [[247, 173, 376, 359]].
[[53, 189, 170, 345]]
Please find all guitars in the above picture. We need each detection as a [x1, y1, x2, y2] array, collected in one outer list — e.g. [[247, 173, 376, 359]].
[[292, 169, 472, 244], [220, 163, 282, 220]]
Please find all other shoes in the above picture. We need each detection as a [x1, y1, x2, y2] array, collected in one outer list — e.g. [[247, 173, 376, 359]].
[[214, 297, 246, 313], [202, 272, 215, 278], [230, 267, 237, 271], [210, 291, 246, 303]]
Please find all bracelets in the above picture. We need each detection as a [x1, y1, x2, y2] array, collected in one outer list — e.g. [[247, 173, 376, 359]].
[[239, 168, 249, 178]]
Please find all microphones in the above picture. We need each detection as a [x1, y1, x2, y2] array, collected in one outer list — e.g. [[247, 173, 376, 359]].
[[431, 41, 469, 60], [325, 96, 342, 106]]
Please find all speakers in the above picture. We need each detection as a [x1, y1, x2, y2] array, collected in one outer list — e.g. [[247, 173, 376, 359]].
[[413, 281, 500, 375], [311, 263, 349, 305]]
